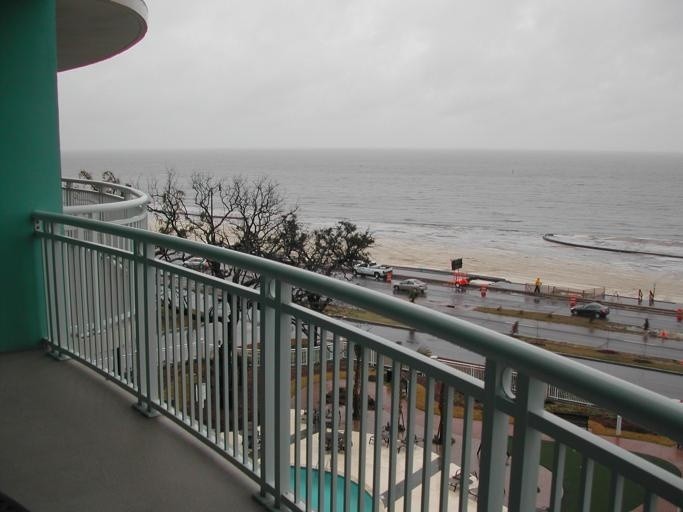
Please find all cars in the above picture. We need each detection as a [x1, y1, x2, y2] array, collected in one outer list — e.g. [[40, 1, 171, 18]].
[[570, 302, 610, 319], [392, 277, 428, 293], [210, 263, 232, 278]]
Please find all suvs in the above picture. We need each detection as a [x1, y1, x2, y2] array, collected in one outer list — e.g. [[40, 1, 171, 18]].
[[154, 247, 179, 262], [182, 256, 208, 272]]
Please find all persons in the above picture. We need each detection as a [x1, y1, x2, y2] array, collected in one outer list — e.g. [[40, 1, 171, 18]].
[[511, 320, 519, 336], [532, 277, 543, 295], [407, 285, 416, 302], [640, 318, 650, 342]]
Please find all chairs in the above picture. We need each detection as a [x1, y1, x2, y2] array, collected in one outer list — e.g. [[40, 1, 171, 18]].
[[303, 407, 353, 454], [469, 487, 505, 502], [448, 469, 473, 492], [368, 430, 416, 454], [242, 422, 261, 459]]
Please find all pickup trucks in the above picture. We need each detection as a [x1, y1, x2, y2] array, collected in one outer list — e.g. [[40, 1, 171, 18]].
[[350, 259, 393, 279]]
